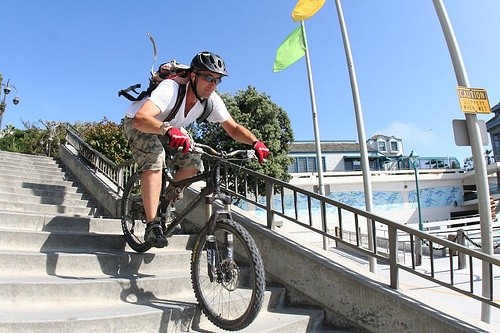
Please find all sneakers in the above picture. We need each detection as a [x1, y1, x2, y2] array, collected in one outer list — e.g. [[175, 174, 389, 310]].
[[163, 206, 181, 230], [144, 221, 168, 247]]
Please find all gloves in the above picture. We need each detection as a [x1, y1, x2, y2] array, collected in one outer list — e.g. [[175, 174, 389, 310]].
[[165, 127, 190, 154], [253, 141, 270, 164]]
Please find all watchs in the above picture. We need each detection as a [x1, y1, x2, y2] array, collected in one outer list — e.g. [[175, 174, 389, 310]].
[[160, 121, 170, 135]]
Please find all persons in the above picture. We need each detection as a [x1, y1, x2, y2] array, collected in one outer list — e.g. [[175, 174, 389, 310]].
[[122, 51, 270, 248]]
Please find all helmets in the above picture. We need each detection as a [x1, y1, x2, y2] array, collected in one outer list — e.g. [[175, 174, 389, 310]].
[[190, 51, 229, 77]]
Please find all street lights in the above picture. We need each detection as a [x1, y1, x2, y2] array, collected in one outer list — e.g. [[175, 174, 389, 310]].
[[0, 77, 20, 138], [37, 119, 62, 158]]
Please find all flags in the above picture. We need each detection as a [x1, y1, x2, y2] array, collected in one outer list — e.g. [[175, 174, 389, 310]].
[[292, 0, 326, 21], [273, 26, 308, 72]]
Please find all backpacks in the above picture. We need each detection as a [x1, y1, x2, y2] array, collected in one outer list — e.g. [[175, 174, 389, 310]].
[[147, 60, 213, 123]]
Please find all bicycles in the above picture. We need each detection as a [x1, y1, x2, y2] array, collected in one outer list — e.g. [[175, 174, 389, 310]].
[[118, 138, 283, 332]]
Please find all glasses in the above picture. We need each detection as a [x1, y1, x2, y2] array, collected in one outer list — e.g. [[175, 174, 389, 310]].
[[193, 71, 221, 85]]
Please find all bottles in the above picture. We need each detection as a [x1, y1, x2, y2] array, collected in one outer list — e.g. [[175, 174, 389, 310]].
[[164, 187, 183, 202]]
